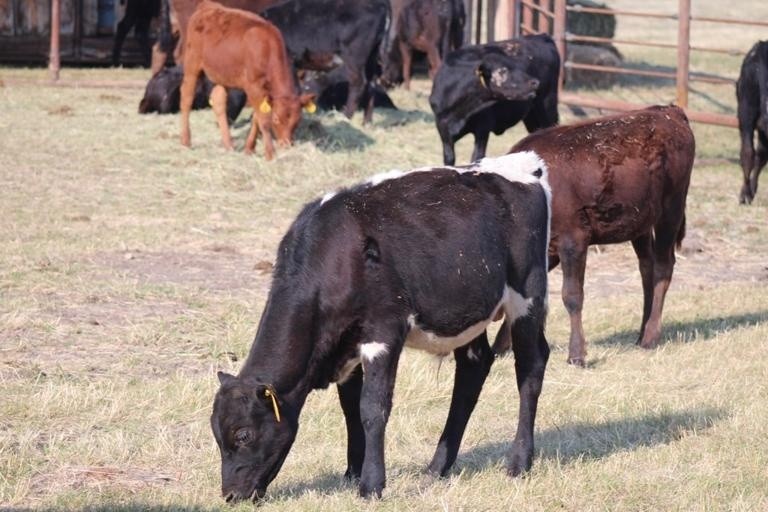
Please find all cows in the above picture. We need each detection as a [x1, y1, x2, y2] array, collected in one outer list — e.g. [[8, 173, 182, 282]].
[[732, 37, 768, 205], [425, 31, 563, 167], [109, 0, 468, 160], [515, 100, 697, 369], [207, 147, 555, 506], [522, 0, 624, 87]]
